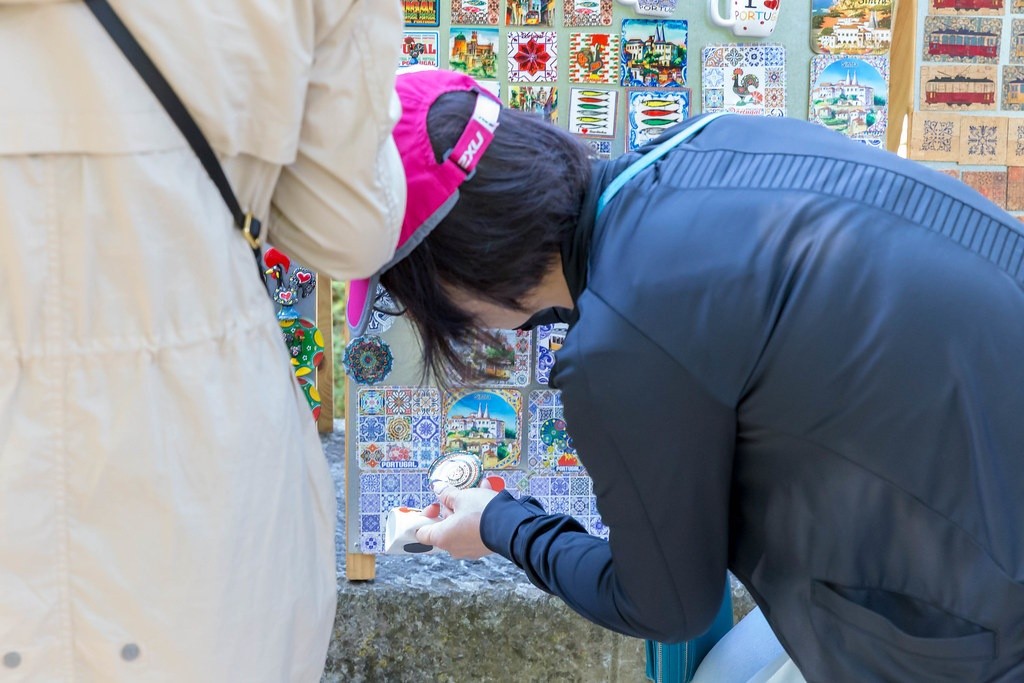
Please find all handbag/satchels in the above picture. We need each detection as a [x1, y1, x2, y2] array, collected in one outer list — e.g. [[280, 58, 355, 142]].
[[646, 572, 734, 683]]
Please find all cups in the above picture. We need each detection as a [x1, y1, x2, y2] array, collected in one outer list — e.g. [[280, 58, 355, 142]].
[[615, 0, 679, 17], [384, 507, 445, 554], [710, 0, 780, 36]]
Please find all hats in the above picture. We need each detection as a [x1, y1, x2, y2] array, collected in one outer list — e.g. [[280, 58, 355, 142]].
[[344, 65, 503, 339]]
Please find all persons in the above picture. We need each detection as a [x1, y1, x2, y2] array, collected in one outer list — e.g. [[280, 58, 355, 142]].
[[0, 0, 407, 683], [348, 63, 1024, 683]]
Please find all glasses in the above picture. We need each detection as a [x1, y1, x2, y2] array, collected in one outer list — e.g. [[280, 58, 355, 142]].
[[373, 284, 407, 317]]
